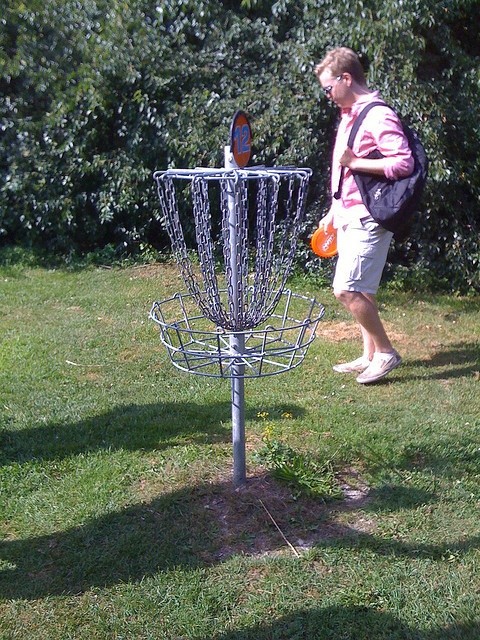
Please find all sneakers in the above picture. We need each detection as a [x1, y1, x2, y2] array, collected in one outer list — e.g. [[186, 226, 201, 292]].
[[333, 357, 369, 373], [356, 351, 403, 383]]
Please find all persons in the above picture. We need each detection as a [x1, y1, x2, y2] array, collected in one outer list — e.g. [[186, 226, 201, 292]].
[[313, 48, 415, 386]]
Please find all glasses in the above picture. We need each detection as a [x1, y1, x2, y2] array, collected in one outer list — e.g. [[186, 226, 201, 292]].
[[319, 77, 342, 95]]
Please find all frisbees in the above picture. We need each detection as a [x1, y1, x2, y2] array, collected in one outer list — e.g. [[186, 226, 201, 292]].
[[310, 225, 338, 257]]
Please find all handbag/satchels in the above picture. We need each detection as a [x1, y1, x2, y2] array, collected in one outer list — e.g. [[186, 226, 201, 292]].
[[353, 137, 428, 228]]
